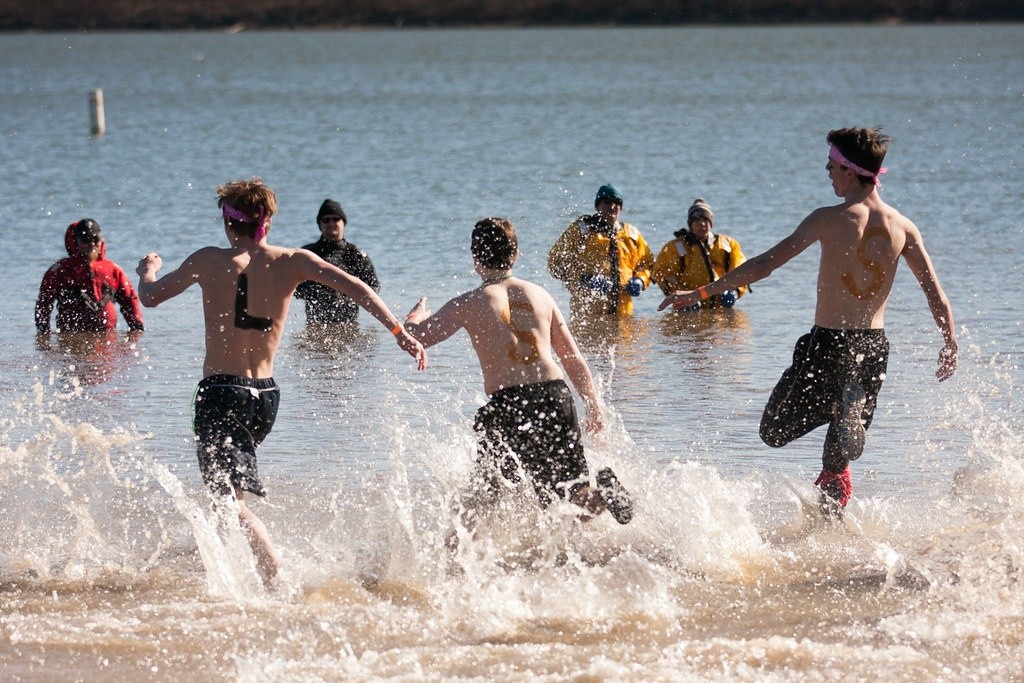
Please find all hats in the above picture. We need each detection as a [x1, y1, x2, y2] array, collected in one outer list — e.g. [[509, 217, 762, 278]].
[[687, 199, 713, 228], [317, 199, 347, 224], [595, 184, 623, 210], [74, 219, 102, 240]]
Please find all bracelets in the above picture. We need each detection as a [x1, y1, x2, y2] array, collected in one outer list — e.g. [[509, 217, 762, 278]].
[[408, 313, 422, 321], [393, 324, 403, 335], [698, 286, 708, 300]]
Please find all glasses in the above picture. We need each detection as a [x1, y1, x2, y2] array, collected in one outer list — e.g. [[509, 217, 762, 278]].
[[321, 217, 341, 224], [79, 237, 100, 245], [602, 198, 621, 205]]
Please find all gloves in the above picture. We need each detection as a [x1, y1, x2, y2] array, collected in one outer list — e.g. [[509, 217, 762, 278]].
[[681, 301, 701, 313], [625, 279, 643, 297], [585, 276, 613, 295], [720, 291, 739, 308]]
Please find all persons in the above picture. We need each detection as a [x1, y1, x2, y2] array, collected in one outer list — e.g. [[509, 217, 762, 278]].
[[293, 199, 380, 322], [405, 218, 633, 524], [650, 199, 748, 314], [658, 128, 957, 520], [135, 180, 427, 500], [34, 219, 144, 331], [548, 184, 654, 315]]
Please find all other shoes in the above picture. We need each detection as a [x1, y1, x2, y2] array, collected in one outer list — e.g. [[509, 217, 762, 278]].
[[595, 468, 636, 524], [835, 383, 866, 461]]
